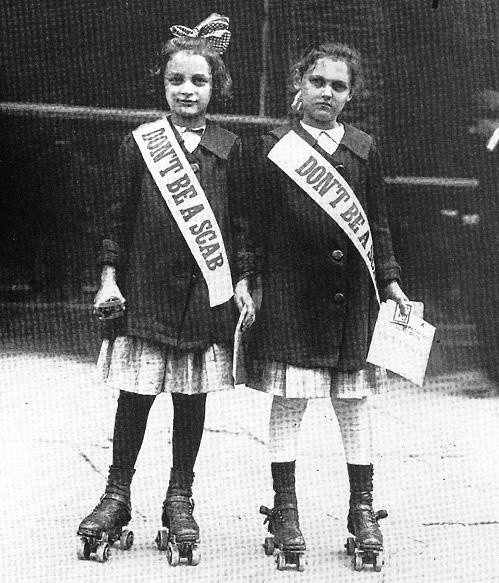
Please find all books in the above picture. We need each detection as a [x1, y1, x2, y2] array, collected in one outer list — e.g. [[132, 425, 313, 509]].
[[366, 299, 439, 386], [230, 304, 253, 385]]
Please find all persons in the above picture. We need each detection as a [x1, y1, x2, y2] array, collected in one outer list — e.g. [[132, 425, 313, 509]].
[[440, 83, 499, 394], [252, 39, 409, 571], [74, 12, 258, 544]]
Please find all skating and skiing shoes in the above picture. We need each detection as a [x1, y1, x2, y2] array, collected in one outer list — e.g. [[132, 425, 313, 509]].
[[260, 501, 306, 571], [77, 491, 133, 563], [156, 496, 200, 565], [346, 504, 388, 571]]
